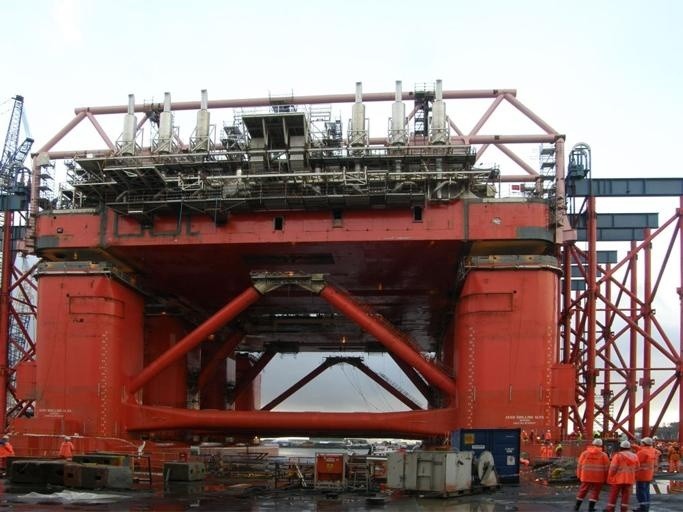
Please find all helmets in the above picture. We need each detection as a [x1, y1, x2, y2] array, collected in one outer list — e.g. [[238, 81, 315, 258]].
[[64, 436, 72, 441], [2, 434, 10, 439], [619, 439, 631, 449], [591, 438, 604, 447], [640, 436, 653, 446]]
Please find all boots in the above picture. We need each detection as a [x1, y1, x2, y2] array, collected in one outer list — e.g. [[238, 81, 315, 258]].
[[618, 503, 629, 512], [631, 502, 651, 512], [574, 500, 583, 511], [587, 501, 598, 512], [602, 503, 617, 512]]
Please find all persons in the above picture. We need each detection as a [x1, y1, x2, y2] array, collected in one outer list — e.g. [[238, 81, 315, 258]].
[[60, 437, 73, 458], [0, 435, 14, 476], [137, 435, 157, 474], [519, 428, 683, 512]]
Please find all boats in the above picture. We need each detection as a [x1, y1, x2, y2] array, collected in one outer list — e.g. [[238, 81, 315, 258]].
[[368, 446, 415, 456]]
